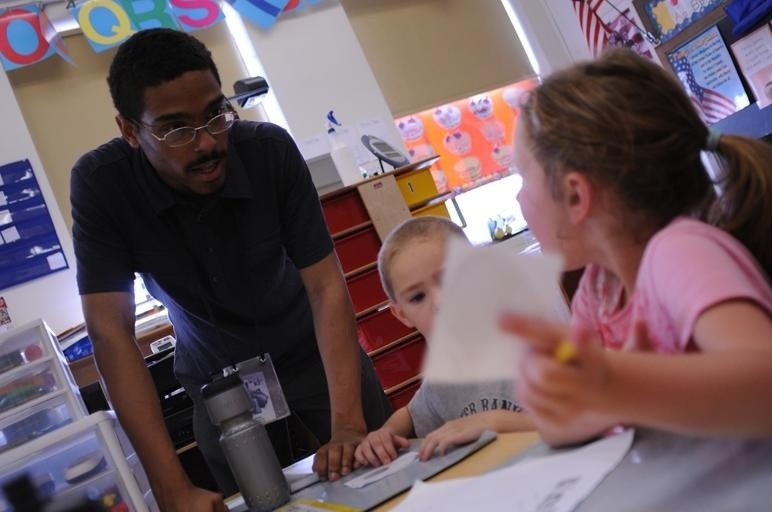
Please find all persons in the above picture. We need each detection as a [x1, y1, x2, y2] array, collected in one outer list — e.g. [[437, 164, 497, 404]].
[[69, 28, 396, 512], [352, 216, 536, 469], [497, 48, 772, 450]]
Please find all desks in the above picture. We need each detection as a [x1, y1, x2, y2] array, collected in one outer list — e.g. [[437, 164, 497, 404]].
[[221, 428, 543, 511]]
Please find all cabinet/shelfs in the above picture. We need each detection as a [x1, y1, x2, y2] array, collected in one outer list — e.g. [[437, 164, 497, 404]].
[[318, 155, 454, 416], [0, 318, 197, 512]]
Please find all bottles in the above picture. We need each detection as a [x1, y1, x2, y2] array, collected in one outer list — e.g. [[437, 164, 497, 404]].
[[199, 369, 291, 512]]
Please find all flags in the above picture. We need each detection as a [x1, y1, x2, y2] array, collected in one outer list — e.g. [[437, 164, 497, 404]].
[[573, 1, 611, 59], [669, 50, 737, 126]]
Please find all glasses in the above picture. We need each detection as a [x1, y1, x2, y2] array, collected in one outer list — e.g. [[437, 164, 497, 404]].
[[121, 97, 237, 147]]
